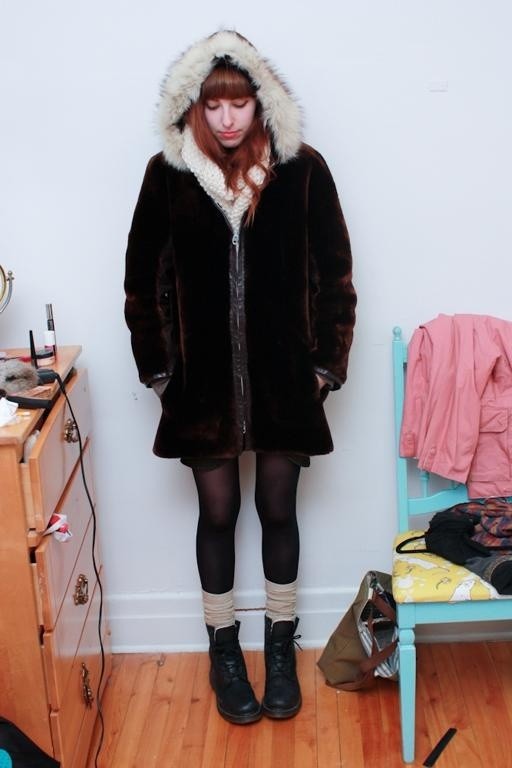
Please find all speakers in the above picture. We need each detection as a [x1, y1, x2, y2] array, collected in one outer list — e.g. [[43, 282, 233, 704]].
[[262, 616, 302, 719], [204, 621, 264, 724]]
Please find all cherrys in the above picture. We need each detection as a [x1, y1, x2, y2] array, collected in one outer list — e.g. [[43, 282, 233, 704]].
[[46, 303, 54, 330], [30, 330, 39, 369]]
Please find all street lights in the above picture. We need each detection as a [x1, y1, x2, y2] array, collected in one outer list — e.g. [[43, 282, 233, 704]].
[[318, 571, 398, 692]]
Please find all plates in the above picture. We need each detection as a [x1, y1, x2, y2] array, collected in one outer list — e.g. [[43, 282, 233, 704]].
[[0, 345, 113, 768]]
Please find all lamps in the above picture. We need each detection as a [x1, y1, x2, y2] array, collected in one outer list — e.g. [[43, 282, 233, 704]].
[[392, 327, 512, 764]]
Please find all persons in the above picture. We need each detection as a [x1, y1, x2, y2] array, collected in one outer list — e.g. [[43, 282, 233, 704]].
[[121, 25, 359, 729]]
